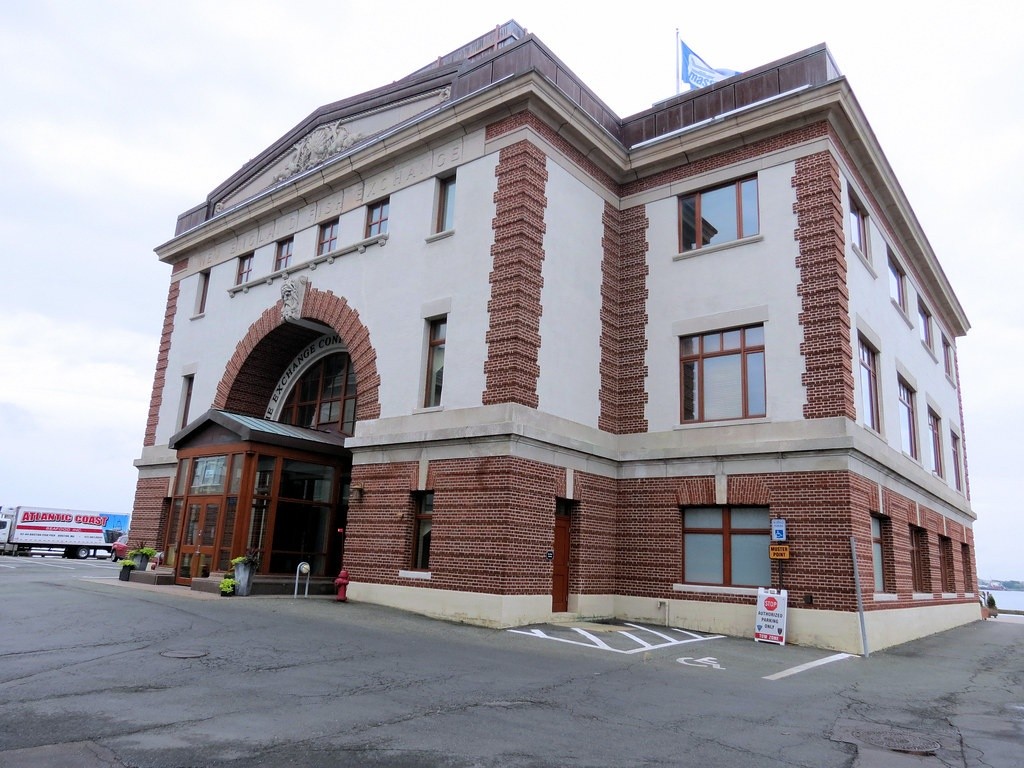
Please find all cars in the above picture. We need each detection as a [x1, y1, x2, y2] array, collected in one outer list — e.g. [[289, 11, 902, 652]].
[[109, 533, 130, 561]]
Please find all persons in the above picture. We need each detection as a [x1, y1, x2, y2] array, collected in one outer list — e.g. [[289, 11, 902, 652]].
[[979, 591, 997, 619]]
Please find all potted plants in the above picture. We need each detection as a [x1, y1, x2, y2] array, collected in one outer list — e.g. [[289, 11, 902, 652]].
[[986, 595, 998, 618], [118, 547, 157, 581], [230, 547, 260, 596], [219, 578, 240, 597]]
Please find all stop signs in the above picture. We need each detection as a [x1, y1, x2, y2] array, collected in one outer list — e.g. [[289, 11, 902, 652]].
[[763, 597, 777, 611]]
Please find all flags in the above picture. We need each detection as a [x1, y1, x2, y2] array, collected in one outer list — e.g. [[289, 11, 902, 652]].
[[681, 40, 743, 90]]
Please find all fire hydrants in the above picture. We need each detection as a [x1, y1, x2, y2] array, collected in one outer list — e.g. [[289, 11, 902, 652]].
[[333, 568, 352, 603]]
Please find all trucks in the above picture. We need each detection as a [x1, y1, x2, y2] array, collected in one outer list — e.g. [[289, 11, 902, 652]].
[[0, 506, 130, 559]]
